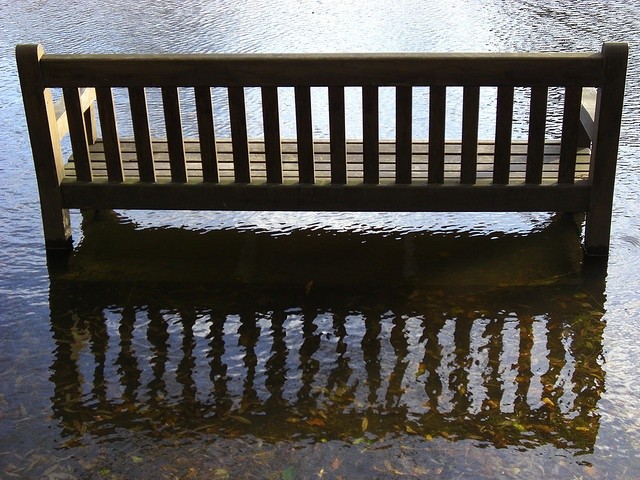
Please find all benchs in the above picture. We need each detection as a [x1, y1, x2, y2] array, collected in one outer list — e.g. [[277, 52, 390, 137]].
[[15, 42, 629, 266], [51, 232, 615, 449]]
